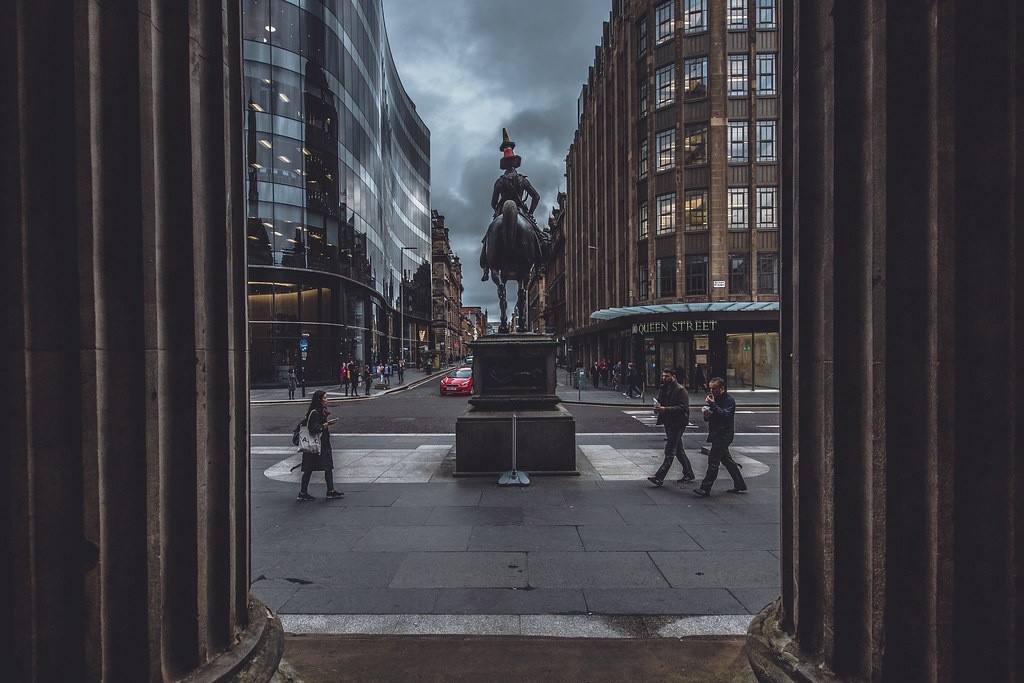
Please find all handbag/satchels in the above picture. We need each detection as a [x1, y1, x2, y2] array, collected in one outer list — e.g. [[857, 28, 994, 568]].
[[298, 409, 323, 455]]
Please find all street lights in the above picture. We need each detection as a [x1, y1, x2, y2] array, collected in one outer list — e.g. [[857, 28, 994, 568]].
[[400, 246, 418, 360]]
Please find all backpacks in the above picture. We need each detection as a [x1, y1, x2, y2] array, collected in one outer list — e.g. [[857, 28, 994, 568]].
[[292, 410, 311, 446]]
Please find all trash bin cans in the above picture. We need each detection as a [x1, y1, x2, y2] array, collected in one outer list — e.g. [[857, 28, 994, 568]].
[[574, 366, 587, 389]]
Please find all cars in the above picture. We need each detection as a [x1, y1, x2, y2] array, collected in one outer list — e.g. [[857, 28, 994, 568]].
[[440, 367, 474, 395]]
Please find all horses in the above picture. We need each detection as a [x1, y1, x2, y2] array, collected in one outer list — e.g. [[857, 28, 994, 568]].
[[485, 200, 537, 333]]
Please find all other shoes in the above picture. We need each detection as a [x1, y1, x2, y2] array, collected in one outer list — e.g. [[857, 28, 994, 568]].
[[636, 394, 640, 397], [677, 475, 695, 484], [726, 488, 748, 494], [648, 477, 663, 486], [694, 488, 710, 496], [326, 490, 344, 498], [296, 492, 315, 501], [640, 393, 643, 398]]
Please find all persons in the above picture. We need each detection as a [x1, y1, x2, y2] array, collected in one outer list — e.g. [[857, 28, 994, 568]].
[[339, 359, 407, 397], [419, 357, 432, 371], [695, 363, 708, 394], [480, 162, 548, 281], [647, 369, 695, 486], [288, 368, 299, 400], [591, 359, 624, 388], [622, 363, 643, 399], [693, 377, 747, 496], [576, 360, 583, 371], [454, 352, 466, 363], [296, 390, 344, 500]]
